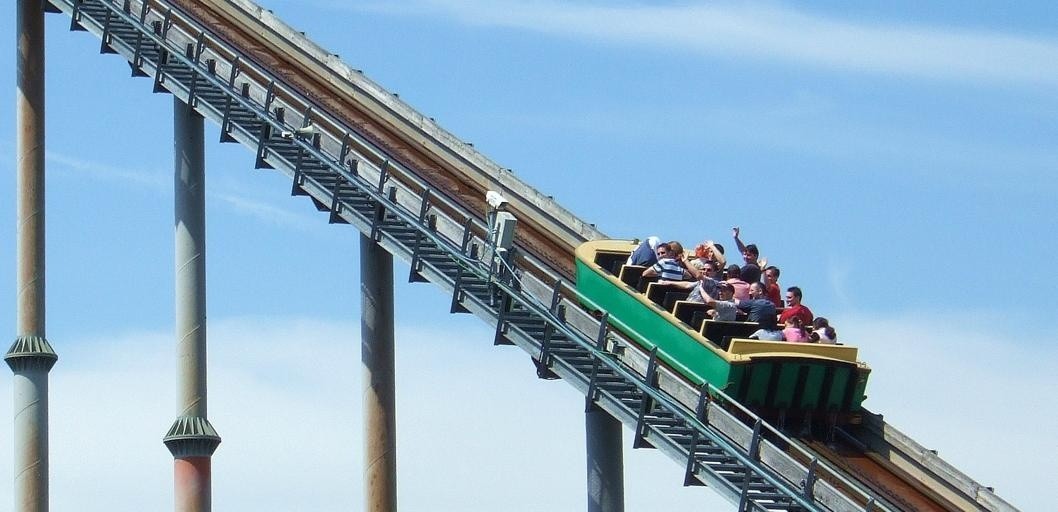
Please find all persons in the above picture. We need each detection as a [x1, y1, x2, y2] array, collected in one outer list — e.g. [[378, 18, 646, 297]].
[[730, 227, 761, 280], [623, 236, 839, 348]]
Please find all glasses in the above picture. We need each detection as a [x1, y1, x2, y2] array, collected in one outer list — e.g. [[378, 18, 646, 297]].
[[703, 268, 712, 272], [657, 252, 666, 255]]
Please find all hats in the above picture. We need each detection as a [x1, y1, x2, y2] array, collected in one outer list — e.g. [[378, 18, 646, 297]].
[[716, 282, 735, 294]]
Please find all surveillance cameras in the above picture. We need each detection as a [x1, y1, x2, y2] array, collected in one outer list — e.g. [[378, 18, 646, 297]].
[[486, 190, 507, 211]]
